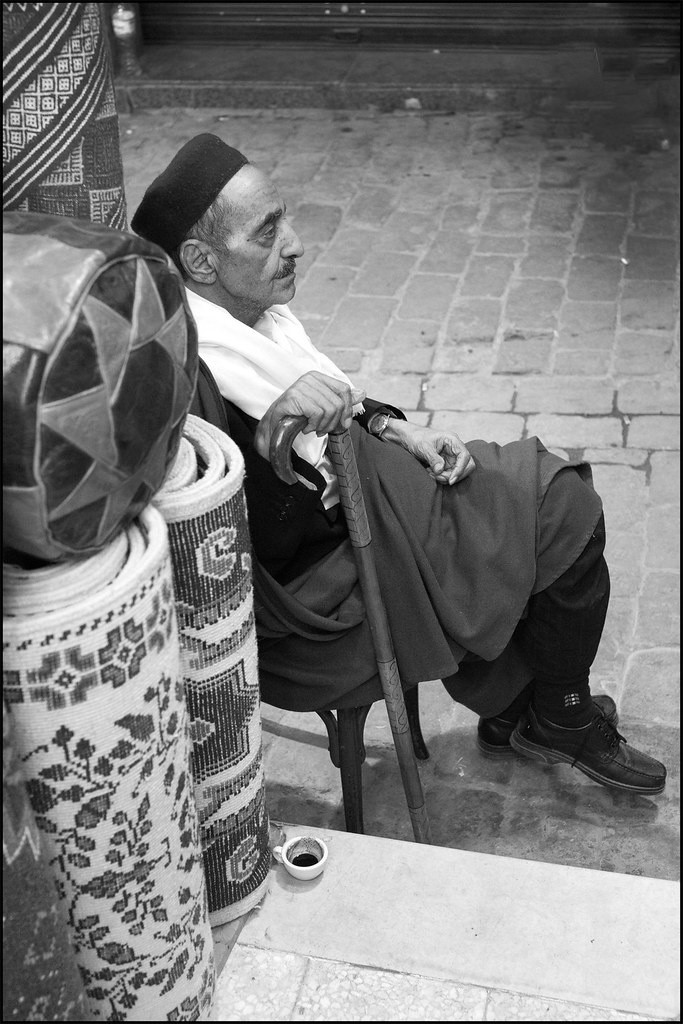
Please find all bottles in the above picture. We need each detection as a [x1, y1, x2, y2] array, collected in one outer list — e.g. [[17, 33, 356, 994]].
[[110, 3, 142, 79]]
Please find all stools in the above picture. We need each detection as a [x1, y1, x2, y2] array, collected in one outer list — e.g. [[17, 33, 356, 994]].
[[190, 340, 430, 834]]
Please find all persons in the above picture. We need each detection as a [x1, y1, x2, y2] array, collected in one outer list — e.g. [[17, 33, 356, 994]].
[[127, 132, 668, 796]]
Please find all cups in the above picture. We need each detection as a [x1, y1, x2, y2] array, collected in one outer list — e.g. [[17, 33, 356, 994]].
[[273, 836, 328, 881]]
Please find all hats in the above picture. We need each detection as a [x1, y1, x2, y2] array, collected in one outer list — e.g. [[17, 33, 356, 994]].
[[130, 133, 249, 254]]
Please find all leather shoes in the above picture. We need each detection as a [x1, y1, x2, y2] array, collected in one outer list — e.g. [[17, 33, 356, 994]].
[[509, 698, 667, 796], [476, 695, 620, 761]]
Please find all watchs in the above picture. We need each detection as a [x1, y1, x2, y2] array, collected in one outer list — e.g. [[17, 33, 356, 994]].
[[367, 414, 394, 436]]
